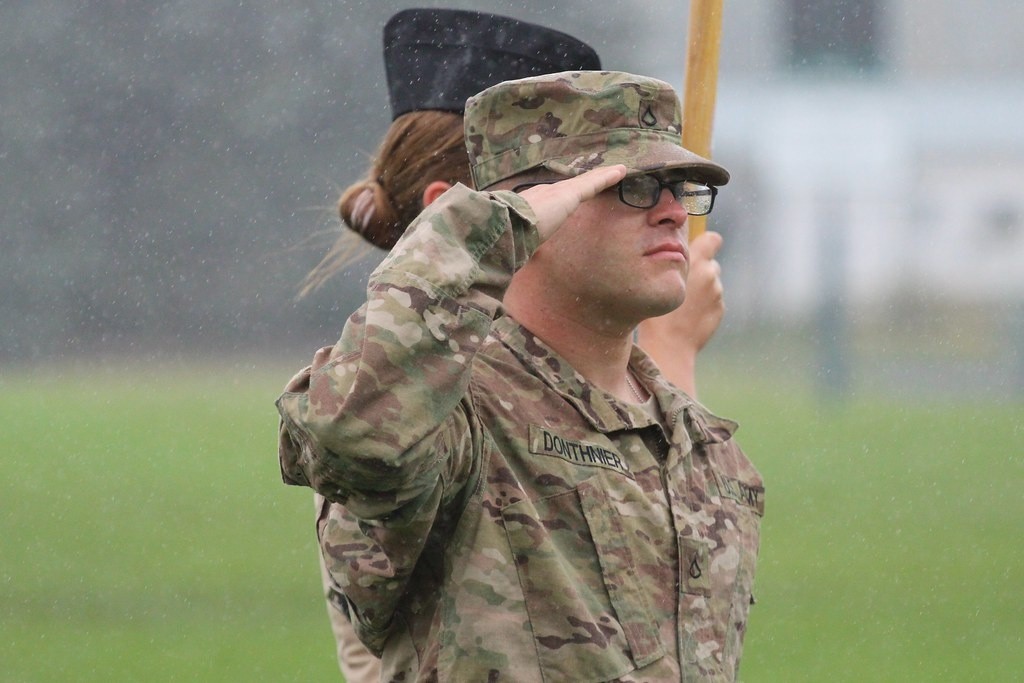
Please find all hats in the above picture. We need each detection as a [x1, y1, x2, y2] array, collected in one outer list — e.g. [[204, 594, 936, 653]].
[[464, 71, 730, 188], [383, 9, 602, 123]]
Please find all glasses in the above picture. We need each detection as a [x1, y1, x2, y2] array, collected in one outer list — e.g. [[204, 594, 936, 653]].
[[512, 175, 718, 216]]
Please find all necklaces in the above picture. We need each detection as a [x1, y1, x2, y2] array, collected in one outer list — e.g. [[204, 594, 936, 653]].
[[626, 377, 645, 403]]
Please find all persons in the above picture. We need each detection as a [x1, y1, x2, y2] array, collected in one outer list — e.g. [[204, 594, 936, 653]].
[[277, 8, 764, 683]]
